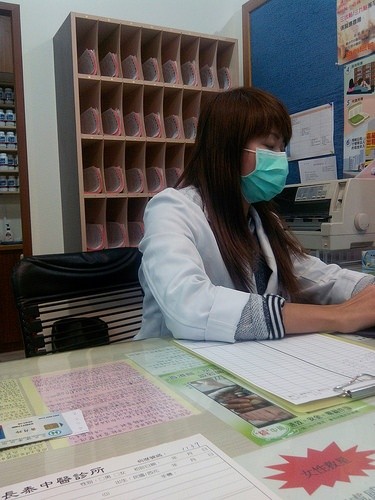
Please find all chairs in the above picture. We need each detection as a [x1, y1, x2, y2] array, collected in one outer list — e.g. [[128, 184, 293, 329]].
[[10, 247, 169, 360]]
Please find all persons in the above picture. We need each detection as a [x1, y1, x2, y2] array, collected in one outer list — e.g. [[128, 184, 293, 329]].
[[133, 87, 375, 343]]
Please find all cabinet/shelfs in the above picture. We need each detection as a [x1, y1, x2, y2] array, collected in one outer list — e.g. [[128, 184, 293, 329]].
[[0, 2, 34, 353], [51, 11, 244, 257]]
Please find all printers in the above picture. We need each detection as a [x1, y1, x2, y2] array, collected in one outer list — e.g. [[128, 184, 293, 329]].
[[266, 177, 375, 250]]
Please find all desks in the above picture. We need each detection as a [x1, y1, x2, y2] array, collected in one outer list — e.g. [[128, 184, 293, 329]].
[[0, 335, 375, 500]]
